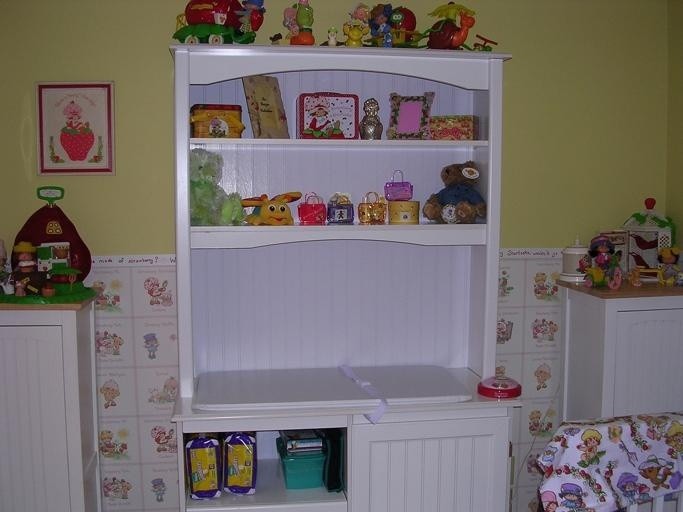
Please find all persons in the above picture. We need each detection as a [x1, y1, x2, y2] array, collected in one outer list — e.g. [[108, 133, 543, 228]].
[[346, 2, 393, 47]]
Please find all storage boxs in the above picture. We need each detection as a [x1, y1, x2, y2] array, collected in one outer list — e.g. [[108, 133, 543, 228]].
[[271, 438, 329, 491]]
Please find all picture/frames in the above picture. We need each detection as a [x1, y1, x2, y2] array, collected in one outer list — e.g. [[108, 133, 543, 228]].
[[34, 80, 117, 176]]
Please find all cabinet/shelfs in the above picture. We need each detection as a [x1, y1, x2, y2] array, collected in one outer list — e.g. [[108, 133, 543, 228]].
[[166, 42, 519, 510], [555, 271, 682, 422], [0, 299, 104, 510]]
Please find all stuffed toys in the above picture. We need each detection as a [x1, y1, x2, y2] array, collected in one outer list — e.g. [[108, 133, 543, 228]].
[[187, 149, 247, 226], [240, 192, 302, 230], [579, 253, 623, 289], [655, 246, 680, 280], [420, 160, 486, 223], [587, 236, 615, 270]]
